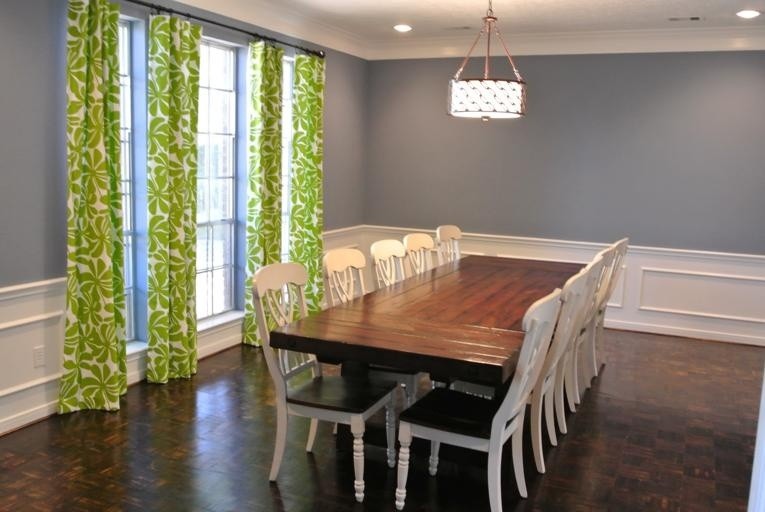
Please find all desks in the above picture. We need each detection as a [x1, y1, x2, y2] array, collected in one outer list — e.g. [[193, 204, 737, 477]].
[[268, 255, 591, 455]]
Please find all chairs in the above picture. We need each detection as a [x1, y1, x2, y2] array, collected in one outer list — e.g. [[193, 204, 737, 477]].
[[386, 286, 562, 512], [252, 262, 399, 502], [324, 246, 417, 436], [403, 232, 435, 276], [370, 239, 407, 290], [527, 236, 629, 475], [435, 223, 462, 266]]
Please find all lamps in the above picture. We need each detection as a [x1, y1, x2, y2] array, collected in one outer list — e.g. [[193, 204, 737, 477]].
[[447, 1, 526, 124]]
